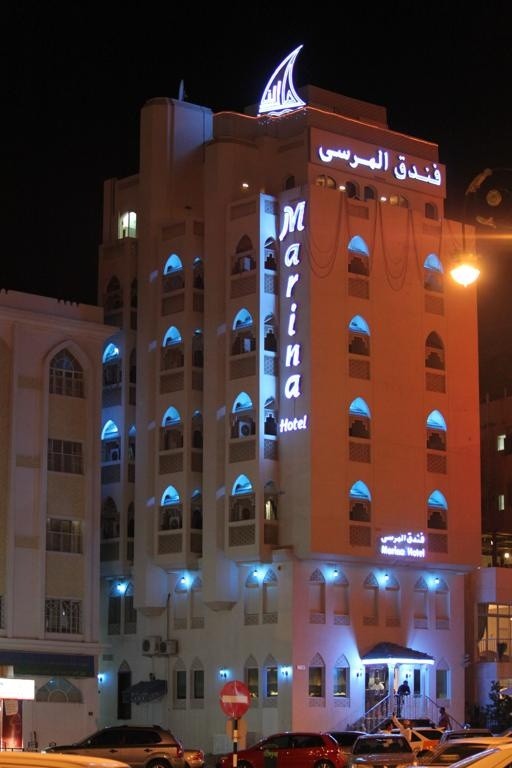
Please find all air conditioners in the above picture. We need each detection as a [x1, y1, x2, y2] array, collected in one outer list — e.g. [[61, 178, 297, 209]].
[[142, 636, 177, 658]]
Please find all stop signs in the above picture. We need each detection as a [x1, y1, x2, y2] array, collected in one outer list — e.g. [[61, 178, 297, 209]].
[[220, 679, 250, 717]]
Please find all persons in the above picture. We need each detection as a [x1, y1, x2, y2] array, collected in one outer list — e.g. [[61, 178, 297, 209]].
[[438, 707, 449, 727], [396, 680, 411, 718]]
